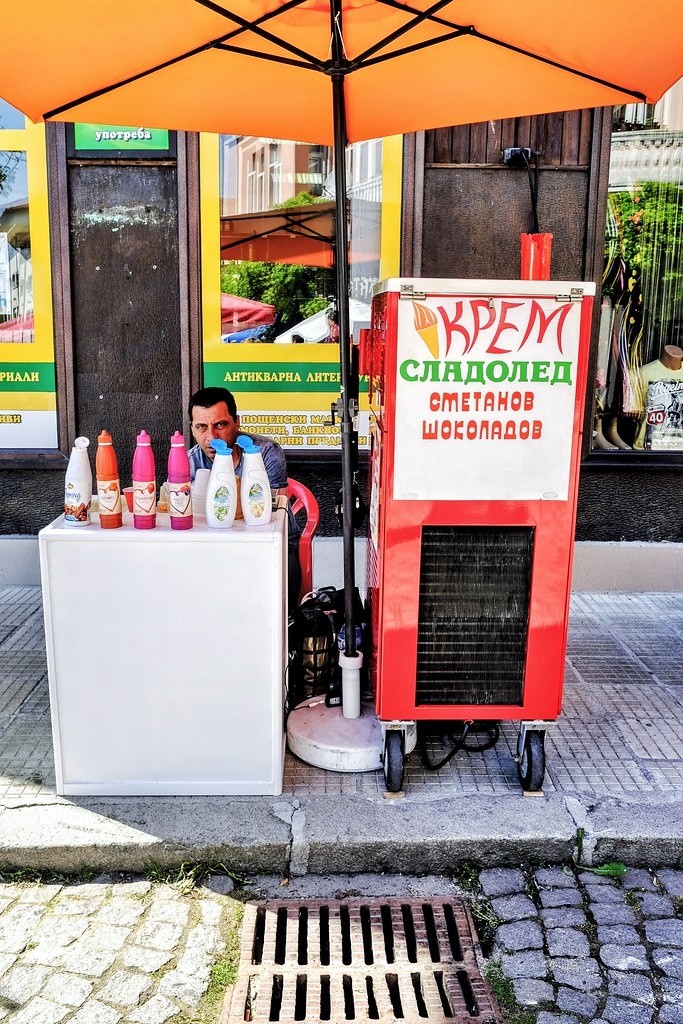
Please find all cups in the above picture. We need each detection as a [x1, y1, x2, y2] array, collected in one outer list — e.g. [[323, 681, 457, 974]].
[[235, 476, 244, 520], [191, 469, 211, 513], [122, 487, 134, 513]]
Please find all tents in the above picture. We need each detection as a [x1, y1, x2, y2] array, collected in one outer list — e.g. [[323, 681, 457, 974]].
[[220, 292, 277, 343]]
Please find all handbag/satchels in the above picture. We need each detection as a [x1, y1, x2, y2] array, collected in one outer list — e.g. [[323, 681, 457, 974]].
[[302, 585, 364, 637]]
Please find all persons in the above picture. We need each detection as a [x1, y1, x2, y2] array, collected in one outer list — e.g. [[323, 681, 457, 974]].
[[292, 308, 341, 343], [186, 387, 302, 608], [594, 190, 683, 451]]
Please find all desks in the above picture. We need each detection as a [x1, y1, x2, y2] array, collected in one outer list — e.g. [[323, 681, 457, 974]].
[[36, 494, 285, 795]]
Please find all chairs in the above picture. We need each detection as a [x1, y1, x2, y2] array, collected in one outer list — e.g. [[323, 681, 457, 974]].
[[282, 477, 319, 603]]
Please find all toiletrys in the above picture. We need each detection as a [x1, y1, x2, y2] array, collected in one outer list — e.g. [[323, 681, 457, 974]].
[[95, 430, 123, 529], [236, 434, 272, 526], [64, 436, 93, 528], [205, 438, 238, 529], [132, 429, 157, 530], [167, 430, 194, 531]]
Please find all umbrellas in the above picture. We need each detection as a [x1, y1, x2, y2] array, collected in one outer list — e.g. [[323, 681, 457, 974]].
[[0, 0, 682, 658], [220, 198, 381, 268]]
